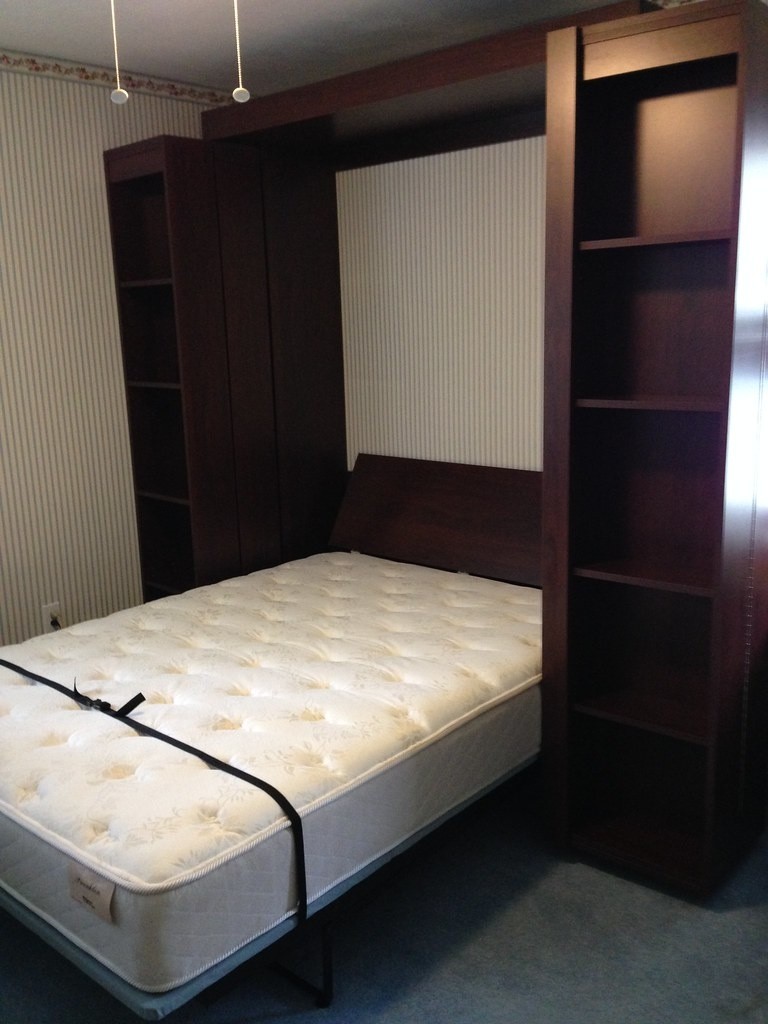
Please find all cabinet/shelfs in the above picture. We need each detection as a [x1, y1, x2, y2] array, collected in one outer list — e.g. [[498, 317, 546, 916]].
[[544, 0, 768, 902], [102, 133, 244, 610]]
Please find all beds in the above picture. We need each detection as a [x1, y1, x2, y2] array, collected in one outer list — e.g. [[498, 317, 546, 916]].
[[1, 451, 540, 1023]]
